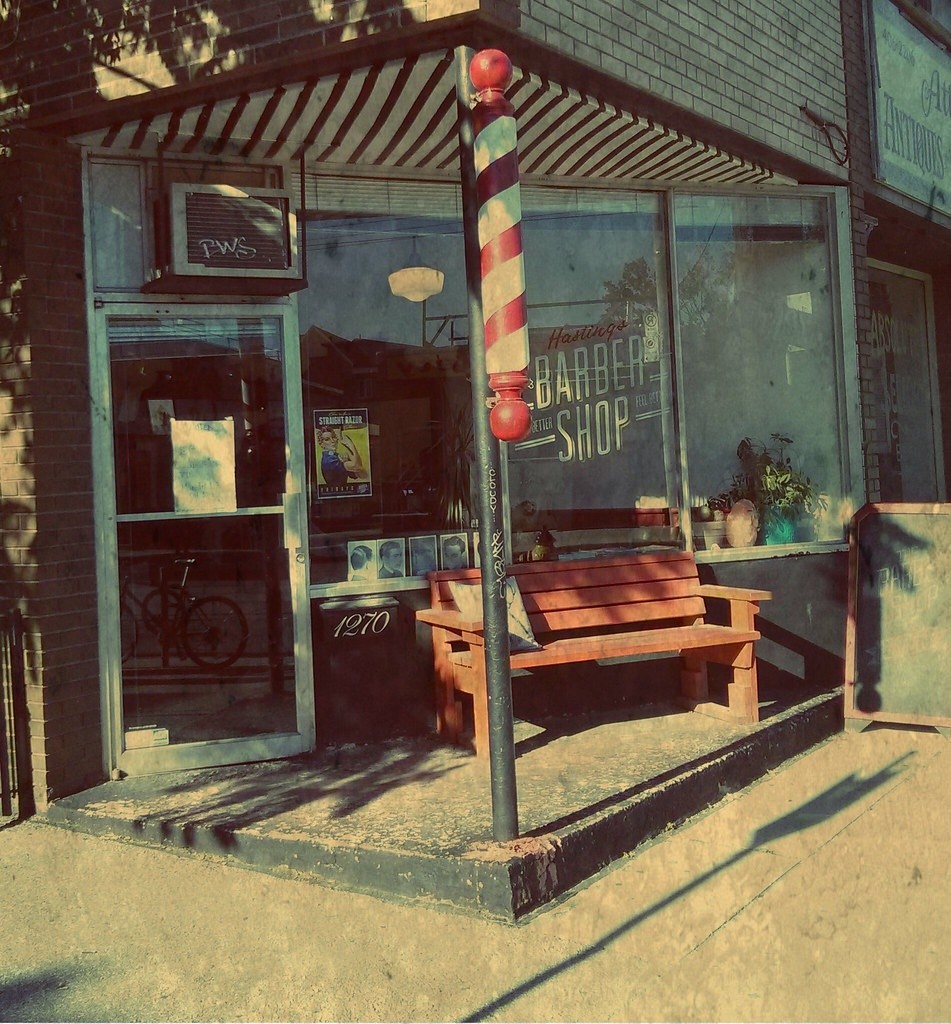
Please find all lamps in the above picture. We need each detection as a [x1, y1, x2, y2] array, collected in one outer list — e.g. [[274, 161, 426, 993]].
[[387, 236, 444, 302]]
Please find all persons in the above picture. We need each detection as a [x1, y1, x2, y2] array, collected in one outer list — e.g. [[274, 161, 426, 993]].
[[351, 546, 375, 580], [444, 536, 468, 570], [317, 427, 368, 485], [379, 541, 404, 579]]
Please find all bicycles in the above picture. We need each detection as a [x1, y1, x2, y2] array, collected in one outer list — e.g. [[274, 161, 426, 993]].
[[121, 555, 249, 668]]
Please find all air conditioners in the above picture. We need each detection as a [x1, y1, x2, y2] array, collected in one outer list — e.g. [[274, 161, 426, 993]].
[[152, 183, 300, 278]]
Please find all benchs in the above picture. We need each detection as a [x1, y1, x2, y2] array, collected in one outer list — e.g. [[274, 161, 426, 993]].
[[415, 551, 773, 762]]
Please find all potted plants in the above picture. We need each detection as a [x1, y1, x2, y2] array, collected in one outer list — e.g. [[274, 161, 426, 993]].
[[691, 506, 725, 550], [706, 434, 811, 546]]
